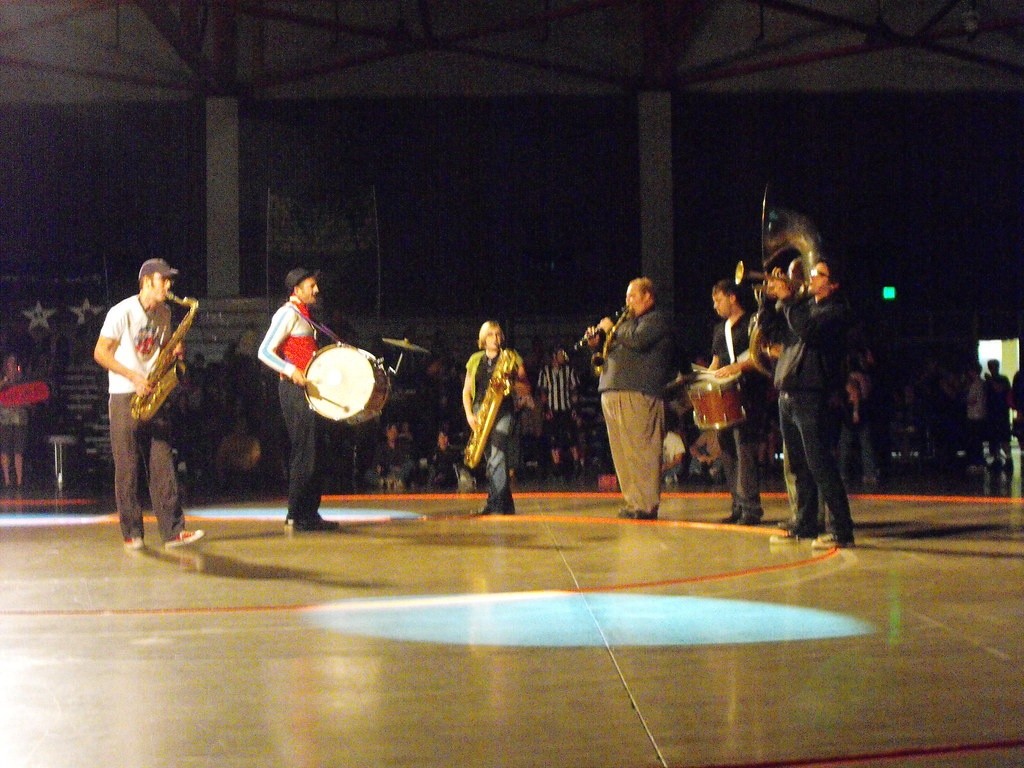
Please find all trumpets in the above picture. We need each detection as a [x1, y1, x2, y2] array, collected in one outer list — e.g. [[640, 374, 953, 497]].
[[734, 261, 809, 298]]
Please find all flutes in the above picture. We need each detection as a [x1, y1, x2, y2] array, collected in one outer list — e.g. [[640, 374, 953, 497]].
[[565, 305, 630, 357]]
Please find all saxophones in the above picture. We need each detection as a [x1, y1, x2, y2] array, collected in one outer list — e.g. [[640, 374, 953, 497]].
[[590, 308, 629, 375], [128, 294, 200, 421], [463, 348, 517, 469]]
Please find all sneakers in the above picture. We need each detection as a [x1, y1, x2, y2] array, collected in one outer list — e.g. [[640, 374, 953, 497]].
[[770, 529, 856, 548], [165, 529, 205, 548], [123, 535, 145, 551]]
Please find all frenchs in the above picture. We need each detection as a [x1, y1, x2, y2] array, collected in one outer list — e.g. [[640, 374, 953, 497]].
[[749, 184, 828, 378]]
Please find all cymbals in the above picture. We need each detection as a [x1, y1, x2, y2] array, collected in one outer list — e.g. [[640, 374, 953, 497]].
[[382, 338, 432, 355]]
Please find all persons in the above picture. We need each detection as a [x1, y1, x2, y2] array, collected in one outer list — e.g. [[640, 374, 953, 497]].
[[675, 278, 764, 525], [0, 322, 1024, 502], [258, 268, 339, 531], [93, 258, 205, 551], [462, 321, 529, 515], [748, 258, 856, 550], [585, 278, 673, 519]]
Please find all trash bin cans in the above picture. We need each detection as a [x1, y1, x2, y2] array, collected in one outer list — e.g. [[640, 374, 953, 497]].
[[41, 436, 81, 494]]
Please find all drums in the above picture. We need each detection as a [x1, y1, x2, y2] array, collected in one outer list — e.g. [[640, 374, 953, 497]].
[[684, 366, 748, 432], [303, 340, 391, 425]]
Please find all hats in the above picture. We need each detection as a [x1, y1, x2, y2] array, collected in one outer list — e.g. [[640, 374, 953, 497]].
[[285, 267, 322, 297], [138, 258, 179, 281]]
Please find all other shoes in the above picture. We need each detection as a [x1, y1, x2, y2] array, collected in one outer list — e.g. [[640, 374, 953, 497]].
[[779, 514, 827, 534], [478, 505, 516, 514], [617, 508, 657, 520], [720, 512, 760, 525], [284, 516, 340, 531]]
